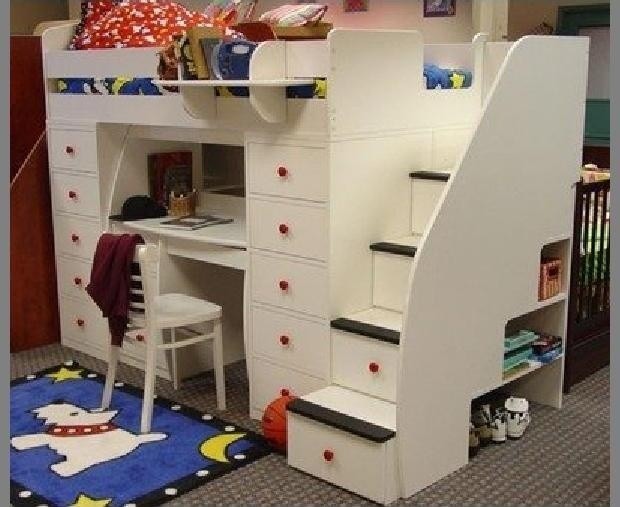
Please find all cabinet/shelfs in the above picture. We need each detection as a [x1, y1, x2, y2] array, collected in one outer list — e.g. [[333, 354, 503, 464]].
[[45, 120, 114, 363], [285, 132, 584, 506], [246, 133, 332, 425]]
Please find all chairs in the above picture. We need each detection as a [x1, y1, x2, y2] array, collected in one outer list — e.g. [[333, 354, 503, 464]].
[[101, 240, 226, 432]]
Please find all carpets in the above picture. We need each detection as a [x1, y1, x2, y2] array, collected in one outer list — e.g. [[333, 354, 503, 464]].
[[9, 362, 275, 507]]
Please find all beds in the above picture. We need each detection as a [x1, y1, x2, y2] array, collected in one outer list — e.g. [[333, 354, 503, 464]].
[[42, 15, 486, 134]]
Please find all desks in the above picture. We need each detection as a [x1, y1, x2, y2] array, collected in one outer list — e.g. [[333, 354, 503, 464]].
[[105, 210, 249, 417]]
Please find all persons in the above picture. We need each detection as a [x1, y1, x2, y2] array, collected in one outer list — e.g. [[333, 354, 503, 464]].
[[160, 212, 235, 231]]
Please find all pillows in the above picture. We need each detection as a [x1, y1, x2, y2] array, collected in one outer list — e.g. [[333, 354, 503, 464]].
[[257, 3, 328, 29]]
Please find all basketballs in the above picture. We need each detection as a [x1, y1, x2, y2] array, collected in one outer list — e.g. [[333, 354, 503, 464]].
[[262, 394, 301, 452]]
